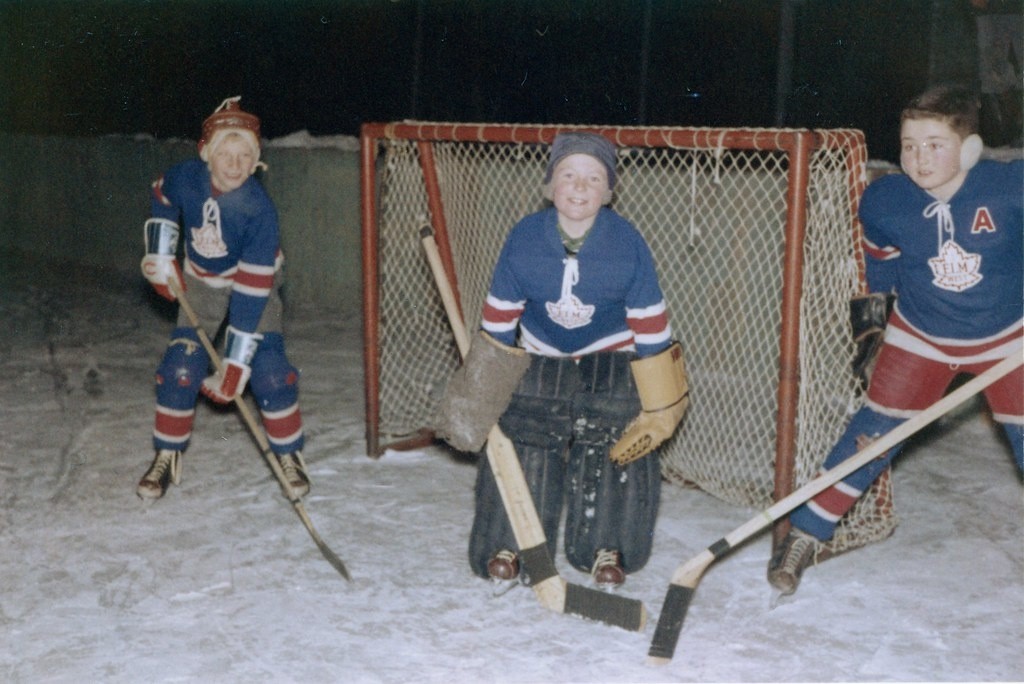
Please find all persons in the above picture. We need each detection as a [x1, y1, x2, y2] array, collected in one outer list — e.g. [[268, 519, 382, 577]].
[[470, 132, 674, 588], [765, 85, 1024, 604], [135, 96, 310, 502]]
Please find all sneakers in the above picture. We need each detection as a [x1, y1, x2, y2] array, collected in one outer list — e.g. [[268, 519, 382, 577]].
[[137, 449, 183, 498], [768, 534, 818, 594], [279, 449, 310, 497]]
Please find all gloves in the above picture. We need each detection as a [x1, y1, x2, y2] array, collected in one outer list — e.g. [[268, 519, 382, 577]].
[[141, 218, 186, 300], [202, 325, 263, 403]]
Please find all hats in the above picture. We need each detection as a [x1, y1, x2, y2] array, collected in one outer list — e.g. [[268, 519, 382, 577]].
[[543, 132, 617, 188], [198, 95, 268, 174]]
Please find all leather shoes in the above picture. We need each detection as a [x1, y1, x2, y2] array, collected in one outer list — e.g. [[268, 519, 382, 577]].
[[591, 547, 625, 586], [488, 550, 518, 581]]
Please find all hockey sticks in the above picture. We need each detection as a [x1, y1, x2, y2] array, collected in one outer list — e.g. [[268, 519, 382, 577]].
[[170, 279, 350, 578], [645, 348, 1023, 670], [419, 225, 650, 636]]
